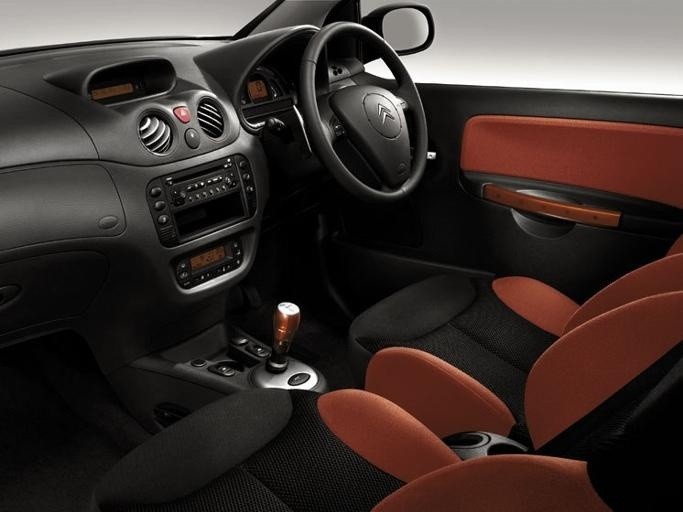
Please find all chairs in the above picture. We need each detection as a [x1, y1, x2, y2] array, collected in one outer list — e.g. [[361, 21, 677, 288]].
[[349, 237, 683, 461], [91, 387, 607, 509]]
